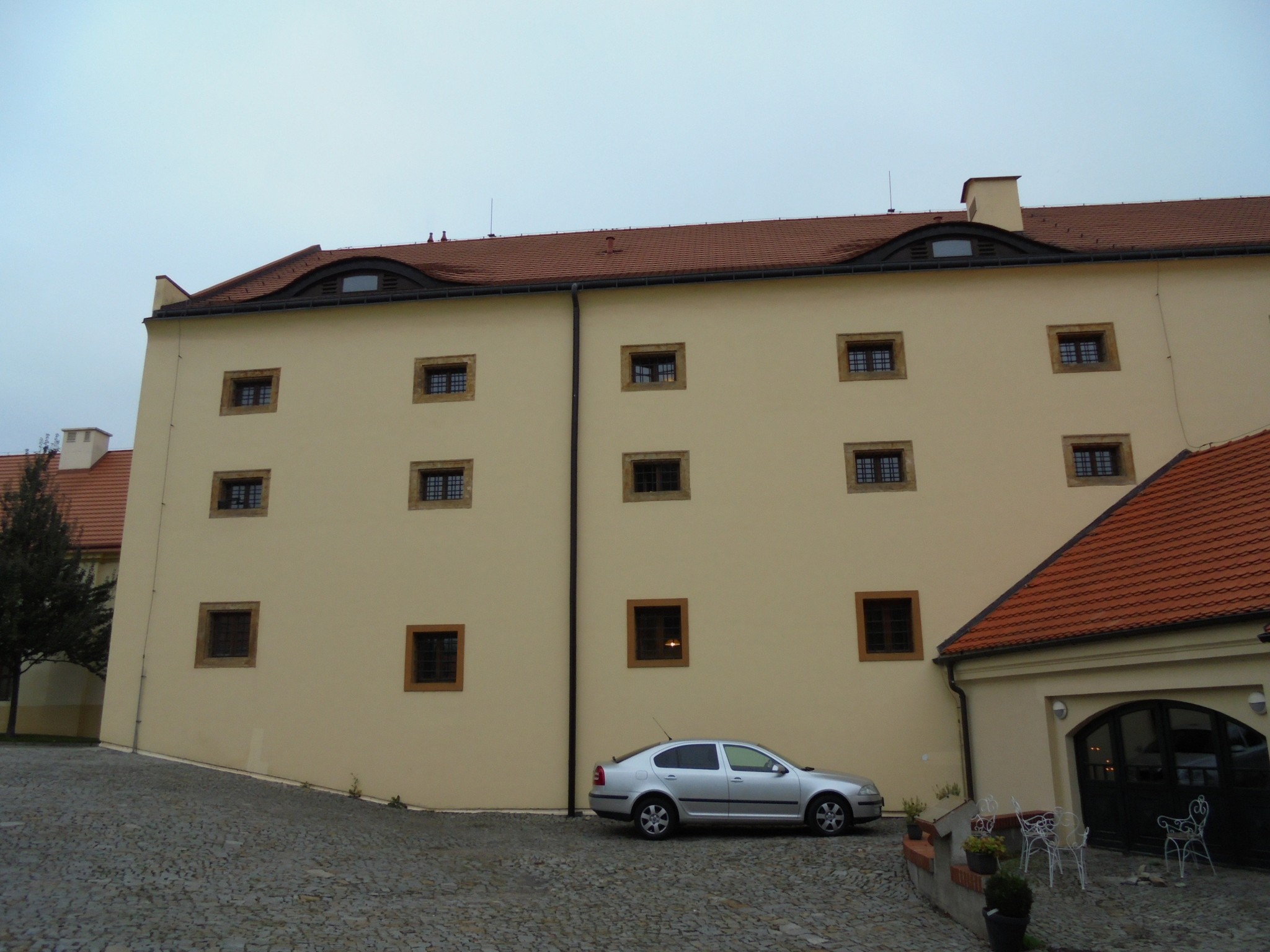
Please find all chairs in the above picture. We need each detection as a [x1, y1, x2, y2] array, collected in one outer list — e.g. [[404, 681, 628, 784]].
[[1010, 794, 1090, 890], [1156, 794, 1217, 879], [970, 794, 1003, 870]]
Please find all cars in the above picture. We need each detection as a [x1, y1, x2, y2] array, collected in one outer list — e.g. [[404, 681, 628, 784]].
[[587, 739, 884, 839]]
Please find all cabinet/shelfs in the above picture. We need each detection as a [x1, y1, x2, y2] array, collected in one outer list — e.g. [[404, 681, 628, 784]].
[[639, 614, 682, 660]]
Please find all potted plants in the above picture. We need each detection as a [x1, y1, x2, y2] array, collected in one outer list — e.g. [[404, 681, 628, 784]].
[[959, 834, 1008, 876], [900, 794, 929, 841], [980, 868, 1037, 952]]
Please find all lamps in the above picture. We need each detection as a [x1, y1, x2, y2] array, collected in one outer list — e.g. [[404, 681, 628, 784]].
[[1053, 701, 1067, 718], [1247, 692, 1267, 714], [663, 637, 682, 659]]
